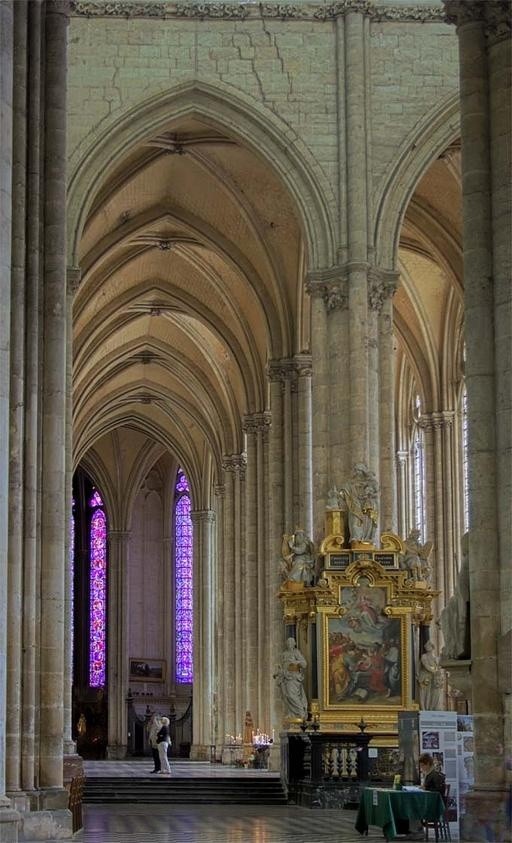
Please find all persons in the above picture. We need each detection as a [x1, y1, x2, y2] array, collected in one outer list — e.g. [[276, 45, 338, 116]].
[[338, 462, 382, 543], [416, 639, 442, 711], [399, 528, 431, 581], [280, 527, 314, 586], [155, 716, 174, 774], [272, 636, 308, 720], [417, 753, 446, 800], [77, 713, 87, 734], [329, 578, 401, 705], [148, 715, 163, 773]]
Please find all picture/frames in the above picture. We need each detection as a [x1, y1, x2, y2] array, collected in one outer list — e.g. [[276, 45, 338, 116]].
[[323, 570, 409, 712], [129, 658, 167, 683]]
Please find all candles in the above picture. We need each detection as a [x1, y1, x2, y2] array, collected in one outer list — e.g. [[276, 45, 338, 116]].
[[252, 728, 275, 744]]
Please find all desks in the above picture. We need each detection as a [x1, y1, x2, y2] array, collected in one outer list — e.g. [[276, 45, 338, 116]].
[[254, 746, 273, 769], [355, 787, 446, 843], [224, 745, 243, 767]]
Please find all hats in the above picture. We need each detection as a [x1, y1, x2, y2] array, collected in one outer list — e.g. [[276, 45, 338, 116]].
[[160, 717, 170, 725]]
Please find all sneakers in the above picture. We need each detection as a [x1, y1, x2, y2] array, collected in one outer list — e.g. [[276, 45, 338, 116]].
[[405, 832, 426, 840], [150, 769, 172, 774]]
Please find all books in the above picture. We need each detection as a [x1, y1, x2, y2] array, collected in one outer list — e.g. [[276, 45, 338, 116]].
[[401, 785, 422, 791]]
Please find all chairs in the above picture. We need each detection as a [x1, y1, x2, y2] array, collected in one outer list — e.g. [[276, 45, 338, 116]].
[[423, 785, 452, 843]]
